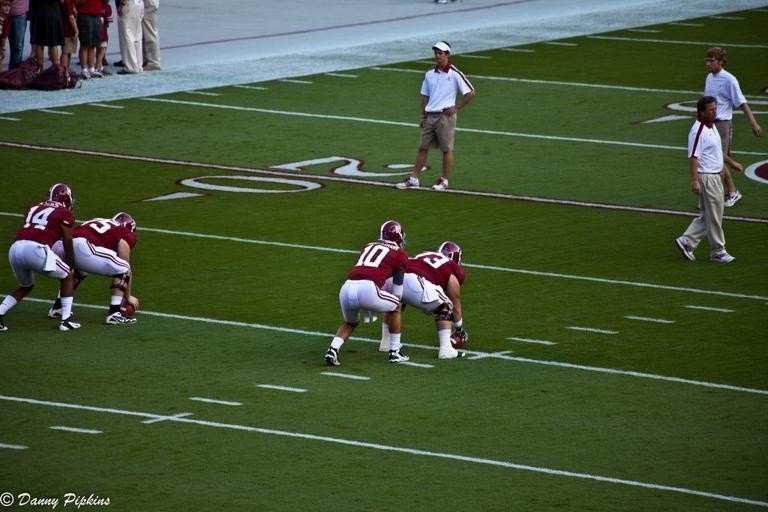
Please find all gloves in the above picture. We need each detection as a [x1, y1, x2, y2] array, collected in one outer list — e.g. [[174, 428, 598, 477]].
[[456, 325, 468, 343]]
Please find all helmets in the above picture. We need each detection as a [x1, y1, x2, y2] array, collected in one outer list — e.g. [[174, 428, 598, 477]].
[[382, 220, 406, 248], [47, 182, 75, 210], [438, 240, 463, 265], [112, 212, 136, 232]]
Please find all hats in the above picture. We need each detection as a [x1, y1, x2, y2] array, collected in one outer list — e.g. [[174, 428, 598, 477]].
[[431, 41, 451, 55]]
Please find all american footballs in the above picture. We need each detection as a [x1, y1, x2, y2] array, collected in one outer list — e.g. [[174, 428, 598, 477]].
[[449, 330, 467, 349], [120, 299, 135, 317]]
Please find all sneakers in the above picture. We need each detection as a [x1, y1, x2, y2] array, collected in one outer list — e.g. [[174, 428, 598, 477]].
[[105, 312, 137, 325], [379, 341, 403, 352], [48, 308, 74, 318], [675, 236, 696, 261], [724, 189, 743, 207], [393, 177, 420, 189], [438, 346, 466, 359], [431, 177, 449, 190], [710, 253, 736, 262], [389, 348, 409, 364], [0, 314, 8, 331], [325, 345, 341, 366], [77, 59, 160, 80], [59, 317, 81, 331]]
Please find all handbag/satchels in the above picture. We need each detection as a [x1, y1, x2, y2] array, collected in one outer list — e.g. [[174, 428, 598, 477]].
[[0, 56, 77, 89]]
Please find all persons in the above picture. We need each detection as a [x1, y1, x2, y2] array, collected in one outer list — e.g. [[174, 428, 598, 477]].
[[1, 1, 162, 82], [392, 41, 476, 192], [699, 46, 761, 207], [1, 178, 81, 333], [45, 203, 139, 326], [677, 97, 737, 264], [322, 219, 408, 365], [379, 238, 469, 361]]
